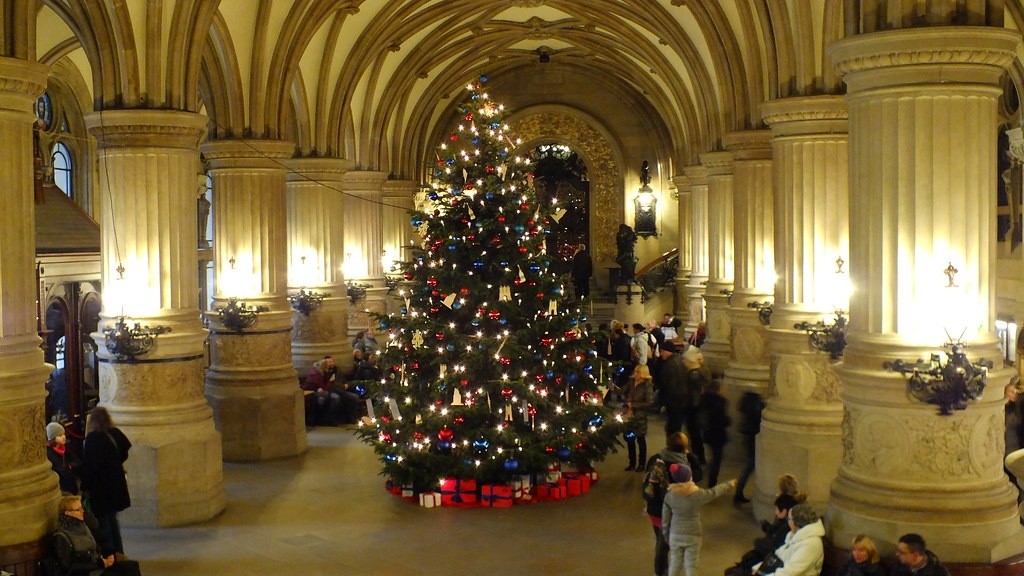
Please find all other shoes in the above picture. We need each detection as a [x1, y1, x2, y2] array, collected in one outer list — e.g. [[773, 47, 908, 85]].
[[733, 495, 750, 503]]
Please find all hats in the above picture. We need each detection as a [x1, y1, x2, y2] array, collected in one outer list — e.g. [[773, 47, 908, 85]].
[[313, 359, 327, 371], [670, 463, 693, 482], [46, 422, 65, 441], [661, 341, 676, 353]]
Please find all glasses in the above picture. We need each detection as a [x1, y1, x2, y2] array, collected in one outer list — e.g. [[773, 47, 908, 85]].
[[66, 506, 81, 512]]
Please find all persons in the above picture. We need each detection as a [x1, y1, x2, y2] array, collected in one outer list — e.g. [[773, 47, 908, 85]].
[[569, 244, 593, 308], [837, 532, 951, 576], [69, 406, 133, 555], [352, 328, 383, 381], [609, 364, 654, 472], [642, 431, 738, 576], [302, 356, 364, 427], [584, 313, 712, 465], [50, 495, 142, 576], [46, 421, 78, 496], [724, 473, 825, 576], [695, 381, 769, 503]]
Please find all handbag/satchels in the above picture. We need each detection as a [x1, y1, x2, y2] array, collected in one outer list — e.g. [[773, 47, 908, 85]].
[[607, 337, 612, 355]]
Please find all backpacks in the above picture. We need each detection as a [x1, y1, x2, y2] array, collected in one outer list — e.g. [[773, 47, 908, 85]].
[[641, 454, 670, 500], [348, 366, 378, 382], [35, 530, 75, 576]]
